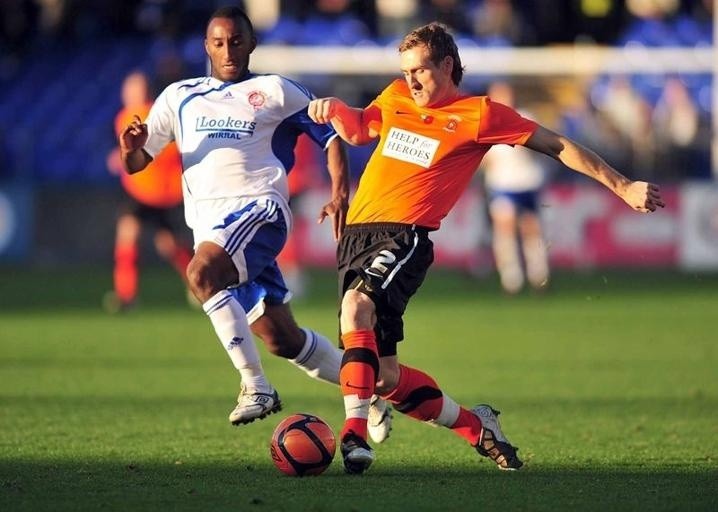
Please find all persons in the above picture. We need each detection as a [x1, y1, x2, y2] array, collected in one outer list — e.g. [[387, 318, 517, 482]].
[[95, 71, 204, 319], [114, 4, 395, 449], [298, 23, 669, 479], [275, 1, 716, 303]]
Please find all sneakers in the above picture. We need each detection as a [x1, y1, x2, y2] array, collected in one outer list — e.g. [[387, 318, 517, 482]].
[[340, 432, 372, 472], [469, 404, 523, 468], [229, 389, 281, 425], [367, 397, 393, 444]]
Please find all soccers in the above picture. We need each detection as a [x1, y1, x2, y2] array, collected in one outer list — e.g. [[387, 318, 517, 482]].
[[269, 413, 337, 478]]
